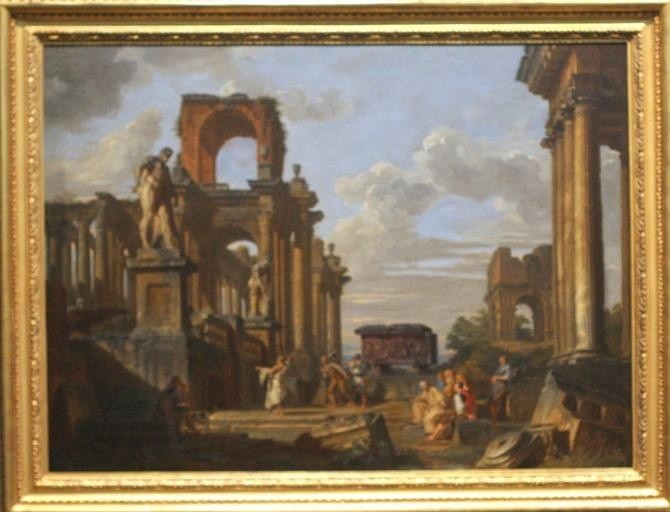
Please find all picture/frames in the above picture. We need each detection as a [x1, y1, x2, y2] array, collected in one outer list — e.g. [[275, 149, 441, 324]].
[[2, 2, 669, 512]]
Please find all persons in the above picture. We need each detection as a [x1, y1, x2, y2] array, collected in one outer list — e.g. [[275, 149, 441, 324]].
[[131, 147, 179, 251], [157, 374, 194, 431], [254, 353, 512, 440]]
